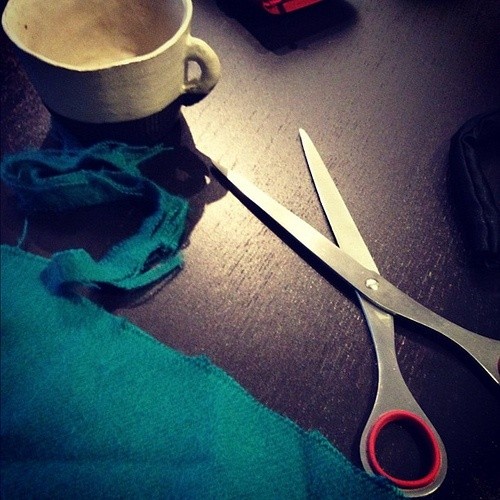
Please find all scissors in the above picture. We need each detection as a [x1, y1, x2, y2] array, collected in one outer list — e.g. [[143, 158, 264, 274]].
[[210, 127, 500, 499]]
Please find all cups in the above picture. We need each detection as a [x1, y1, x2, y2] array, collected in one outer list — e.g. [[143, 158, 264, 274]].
[[2, 0, 222, 125]]
